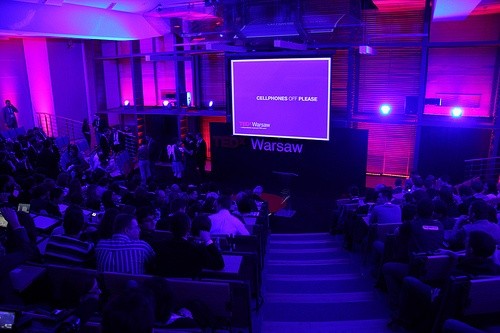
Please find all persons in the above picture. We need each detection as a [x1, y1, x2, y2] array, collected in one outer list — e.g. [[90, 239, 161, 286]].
[[0, 99, 267, 333], [350, 171, 500, 333]]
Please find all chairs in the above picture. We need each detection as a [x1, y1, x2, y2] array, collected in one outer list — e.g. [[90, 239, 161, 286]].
[[0, 127, 267, 333], [333, 176, 500, 333]]
